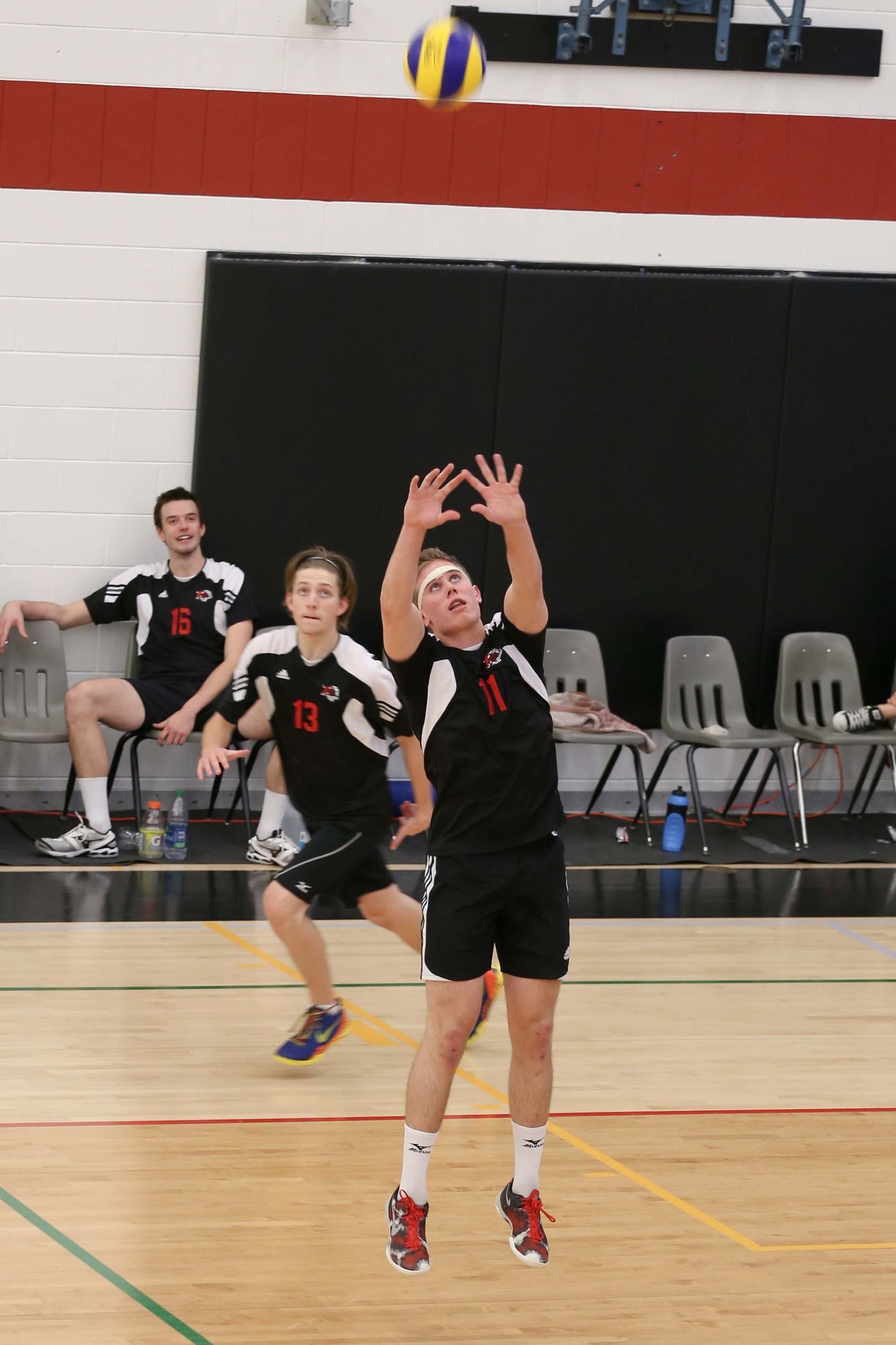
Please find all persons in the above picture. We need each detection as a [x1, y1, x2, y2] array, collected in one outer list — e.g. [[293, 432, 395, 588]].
[[0, 486, 303, 868], [834, 687, 896, 733], [381, 453, 569, 1274], [231, 546, 506, 1067]]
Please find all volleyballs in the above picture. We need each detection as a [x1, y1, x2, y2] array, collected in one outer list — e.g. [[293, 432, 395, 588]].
[[403, 17, 487, 101]]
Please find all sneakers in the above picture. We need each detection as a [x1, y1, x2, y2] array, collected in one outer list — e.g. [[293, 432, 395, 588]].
[[35, 811, 120, 859], [494, 1177, 557, 1268], [246, 828, 302, 867], [832, 704, 884, 736], [465, 964, 505, 1049], [383, 1184, 433, 1274], [267, 997, 352, 1065]]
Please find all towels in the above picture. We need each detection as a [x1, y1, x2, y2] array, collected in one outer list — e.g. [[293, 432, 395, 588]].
[[550, 690, 659, 755]]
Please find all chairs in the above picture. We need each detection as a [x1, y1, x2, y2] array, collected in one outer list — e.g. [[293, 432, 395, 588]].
[[744, 632, 896, 849], [628, 636, 800, 855], [107, 622, 253, 844], [543, 629, 653, 846], [0, 620, 76, 820]]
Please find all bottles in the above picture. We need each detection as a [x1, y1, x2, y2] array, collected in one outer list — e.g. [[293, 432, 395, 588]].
[[117, 828, 140, 850], [138, 800, 165, 859], [662, 786, 689, 852], [165, 790, 188, 860]]
